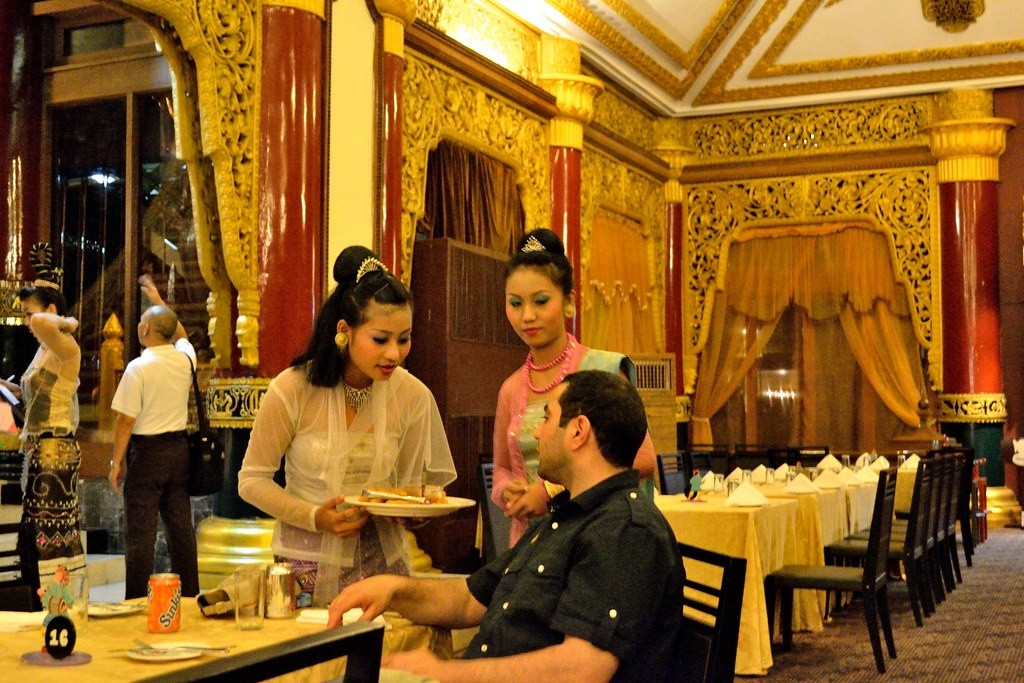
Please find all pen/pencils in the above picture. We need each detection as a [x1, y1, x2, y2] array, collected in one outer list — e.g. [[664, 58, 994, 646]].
[[7, 375, 15, 382]]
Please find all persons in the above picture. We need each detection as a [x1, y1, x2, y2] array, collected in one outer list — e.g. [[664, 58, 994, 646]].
[[108, 279, 200, 600], [491, 228, 657, 548], [0, 271, 83, 611], [237, 245, 458, 608], [327, 368, 685, 683]]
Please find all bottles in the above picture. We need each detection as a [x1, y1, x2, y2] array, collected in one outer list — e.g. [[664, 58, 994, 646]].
[[266, 562, 299, 619]]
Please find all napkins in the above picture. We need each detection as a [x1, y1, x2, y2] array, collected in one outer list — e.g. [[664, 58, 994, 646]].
[[297, 597, 391, 632]]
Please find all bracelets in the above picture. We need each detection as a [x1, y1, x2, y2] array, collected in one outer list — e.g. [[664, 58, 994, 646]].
[[111, 460, 122, 467]]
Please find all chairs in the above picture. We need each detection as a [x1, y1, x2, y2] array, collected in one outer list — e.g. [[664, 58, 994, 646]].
[[476, 445, 988, 683]]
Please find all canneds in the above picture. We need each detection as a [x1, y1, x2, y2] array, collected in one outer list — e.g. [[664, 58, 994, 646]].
[[146, 573, 181, 633]]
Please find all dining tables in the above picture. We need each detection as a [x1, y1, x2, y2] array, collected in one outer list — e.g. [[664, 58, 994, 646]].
[[656, 464, 925, 674], [0, 571, 475, 683]]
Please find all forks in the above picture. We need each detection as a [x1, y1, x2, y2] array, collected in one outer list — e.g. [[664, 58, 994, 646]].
[[136, 641, 231, 651]]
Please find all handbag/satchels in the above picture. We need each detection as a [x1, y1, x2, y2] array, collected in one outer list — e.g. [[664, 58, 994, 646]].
[[187, 429, 225, 496]]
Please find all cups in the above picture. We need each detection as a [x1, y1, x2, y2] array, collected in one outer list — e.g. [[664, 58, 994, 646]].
[[712, 451, 910, 496], [233, 568, 266, 629]]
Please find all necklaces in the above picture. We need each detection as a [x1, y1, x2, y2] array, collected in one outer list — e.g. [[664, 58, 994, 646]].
[[525, 333, 572, 392], [344, 381, 374, 414]]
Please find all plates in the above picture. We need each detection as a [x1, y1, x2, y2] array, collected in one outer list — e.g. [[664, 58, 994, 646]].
[[129, 644, 203, 661], [89, 605, 148, 617], [343, 495, 476, 516]]
